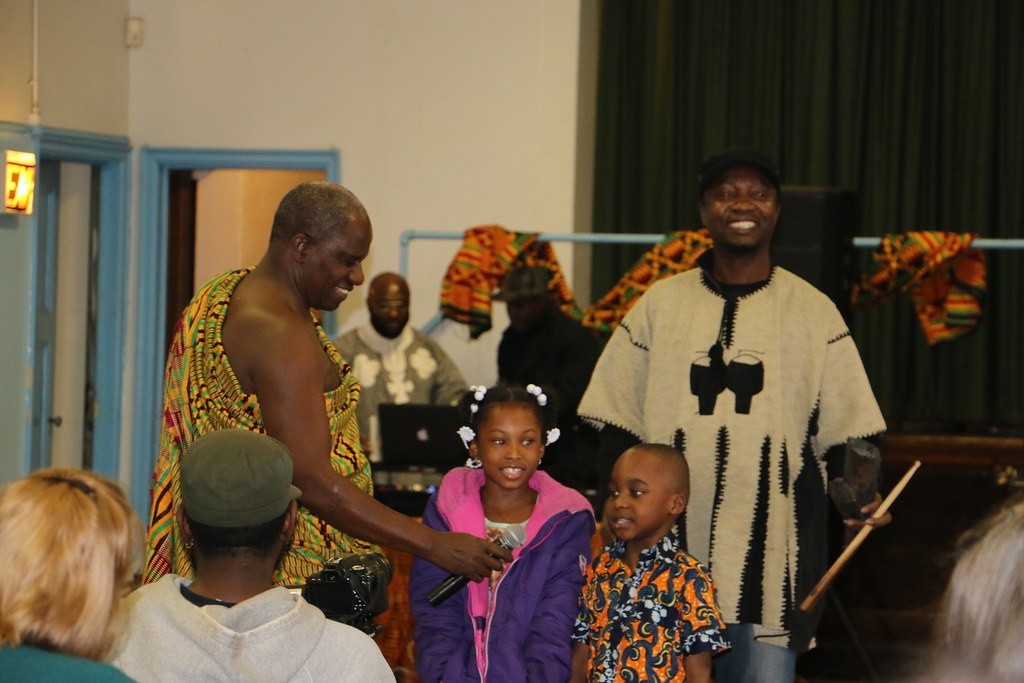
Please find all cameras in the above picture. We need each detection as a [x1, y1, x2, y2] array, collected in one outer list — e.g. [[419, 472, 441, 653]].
[[300, 551, 393, 638]]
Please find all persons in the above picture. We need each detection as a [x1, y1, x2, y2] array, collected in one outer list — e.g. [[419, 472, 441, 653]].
[[568, 444, 731, 683], [330, 272, 471, 461], [497, 266, 613, 413], [144, 181, 513, 587], [908, 491, 1023, 683], [96, 429, 398, 683], [408, 383, 595, 683], [575, 148, 891, 683], [0, 469, 145, 683]]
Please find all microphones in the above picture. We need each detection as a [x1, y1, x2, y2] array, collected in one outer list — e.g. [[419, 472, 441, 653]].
[[426, 523, 527, 608]]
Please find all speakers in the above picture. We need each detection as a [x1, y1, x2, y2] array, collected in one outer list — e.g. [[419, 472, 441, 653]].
[[770, 185, 858, 323]]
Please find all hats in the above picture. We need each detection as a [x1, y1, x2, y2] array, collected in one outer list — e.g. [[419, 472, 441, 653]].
[[489, 266, 558, 302], [181, 428, 302, 528], [697, 146, 782, 190]]
